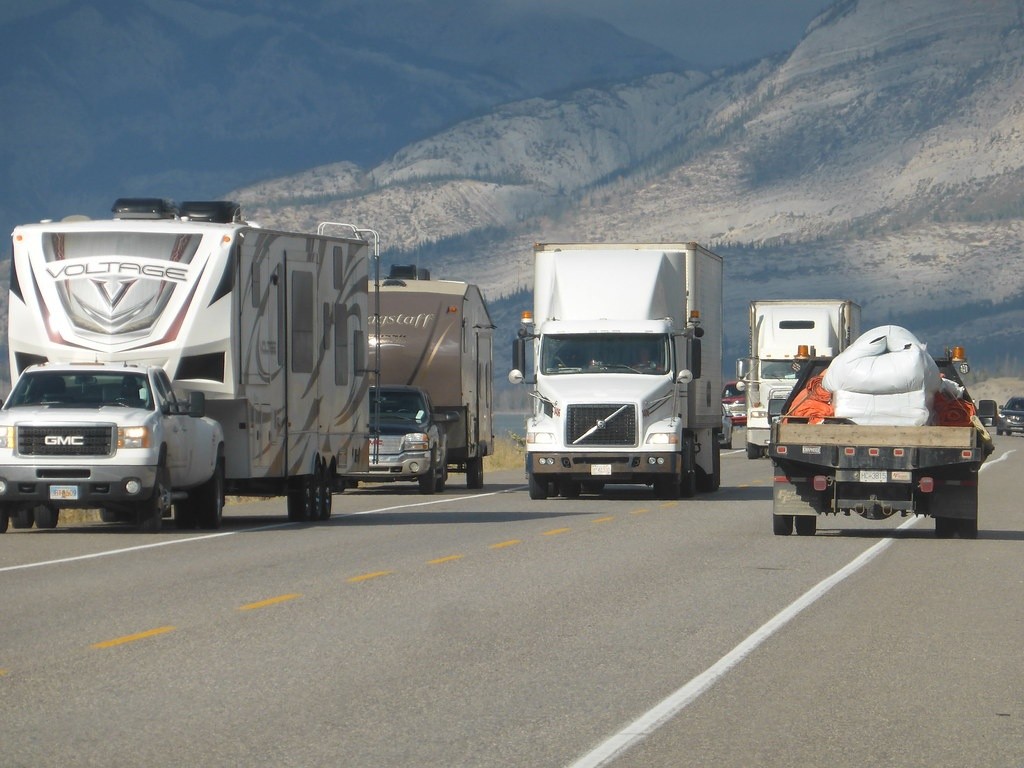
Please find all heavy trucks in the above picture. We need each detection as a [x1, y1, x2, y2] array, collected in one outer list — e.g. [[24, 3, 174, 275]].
[[506, 241, 723, 500], [734, 299, 862, 459]]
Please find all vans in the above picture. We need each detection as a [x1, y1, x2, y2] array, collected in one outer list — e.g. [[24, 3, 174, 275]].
[[721, 380, 748, 425]]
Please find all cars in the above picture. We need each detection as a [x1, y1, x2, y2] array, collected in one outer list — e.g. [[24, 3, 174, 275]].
[[716, 403, 734, 448], [997, 394, 1024, 437]]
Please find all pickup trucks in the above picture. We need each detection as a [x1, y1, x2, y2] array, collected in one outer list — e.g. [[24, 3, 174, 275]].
[[0, 361, 225, 531], [332, 386, 449, 493]]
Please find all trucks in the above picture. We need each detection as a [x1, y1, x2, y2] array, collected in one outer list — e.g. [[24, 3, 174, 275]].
[[766, 339, 996, 537]]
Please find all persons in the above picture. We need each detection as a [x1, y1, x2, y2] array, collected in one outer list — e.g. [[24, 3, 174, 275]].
[[118, 377, 144, 407], [631, 346, 662, 371]]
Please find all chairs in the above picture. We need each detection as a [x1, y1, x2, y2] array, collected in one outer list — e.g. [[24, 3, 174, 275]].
[[28, 375, 143, 407]]
